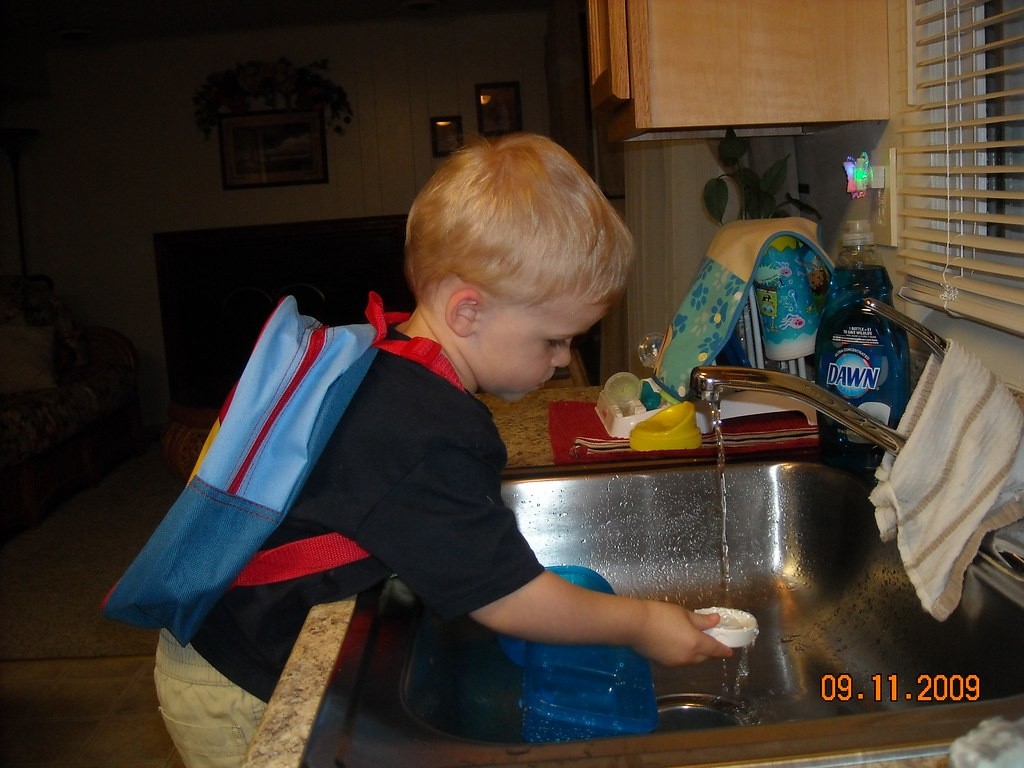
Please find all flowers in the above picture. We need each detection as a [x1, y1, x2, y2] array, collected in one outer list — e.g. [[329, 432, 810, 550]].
[[193, 56, 352, 139]]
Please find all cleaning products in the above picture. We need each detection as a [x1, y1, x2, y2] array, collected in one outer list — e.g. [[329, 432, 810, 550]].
[[813, 218, 912, 471]]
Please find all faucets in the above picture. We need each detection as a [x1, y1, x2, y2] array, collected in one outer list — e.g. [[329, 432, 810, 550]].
[[688, 295, 951, 458]]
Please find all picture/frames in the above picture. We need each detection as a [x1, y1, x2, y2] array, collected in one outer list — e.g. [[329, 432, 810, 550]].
[[430, 116, 463, 157], [217, 106, 329, 189], [475, 80, 523, 136]]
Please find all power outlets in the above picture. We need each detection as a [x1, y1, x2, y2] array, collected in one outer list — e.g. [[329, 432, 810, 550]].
[[871, 147, 897, 247]]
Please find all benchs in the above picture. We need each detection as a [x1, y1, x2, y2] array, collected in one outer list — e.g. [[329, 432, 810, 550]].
[[0, 274, 138, 470]]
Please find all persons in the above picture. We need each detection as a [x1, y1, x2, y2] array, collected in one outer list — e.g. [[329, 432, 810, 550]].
[[153, 132, 731, 768]]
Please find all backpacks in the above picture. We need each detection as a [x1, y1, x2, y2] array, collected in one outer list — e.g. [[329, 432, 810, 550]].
[[100, 295, 466, 650]]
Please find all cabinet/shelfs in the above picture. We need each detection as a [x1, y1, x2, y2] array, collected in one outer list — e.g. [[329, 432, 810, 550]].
[[153, 215, 416, 408], [543, 0, 895, 386]]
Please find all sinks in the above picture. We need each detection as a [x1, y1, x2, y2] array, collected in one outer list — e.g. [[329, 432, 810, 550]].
[[336, 446, 1024, 768]]
